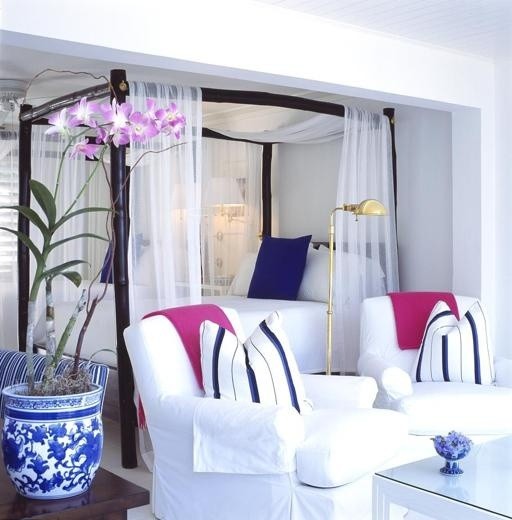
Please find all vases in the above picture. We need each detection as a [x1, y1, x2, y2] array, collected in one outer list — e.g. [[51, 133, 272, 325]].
[[1, 381, 104, 500], [436, 450, 471, 475]]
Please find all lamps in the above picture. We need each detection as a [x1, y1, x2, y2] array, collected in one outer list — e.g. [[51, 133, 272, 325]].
[[326, 199, 388, 375]]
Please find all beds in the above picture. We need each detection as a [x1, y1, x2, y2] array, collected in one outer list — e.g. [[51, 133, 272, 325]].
[[17, 69, 399, 469]]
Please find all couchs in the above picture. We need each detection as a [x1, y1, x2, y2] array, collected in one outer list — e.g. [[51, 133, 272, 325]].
[[0, 350, 110, 420]]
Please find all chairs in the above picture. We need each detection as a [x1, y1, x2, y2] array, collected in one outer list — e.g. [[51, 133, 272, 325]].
[[358, 291, 512, 446], [122, 304, 438, 520]]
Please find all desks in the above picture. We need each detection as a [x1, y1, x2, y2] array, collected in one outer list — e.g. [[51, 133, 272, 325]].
[[0, 449, 150, 520], [371, 435, 512, 520]]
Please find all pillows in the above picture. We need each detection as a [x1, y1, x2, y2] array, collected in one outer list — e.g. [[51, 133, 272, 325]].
[[199, 310, 314, 416], [411, 299, 495, 385], [227, 234, 385, 307]]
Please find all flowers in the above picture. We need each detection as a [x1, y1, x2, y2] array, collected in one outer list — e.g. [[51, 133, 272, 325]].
[[430, 430, 471, 448], [0, 97, 186, 395]]
